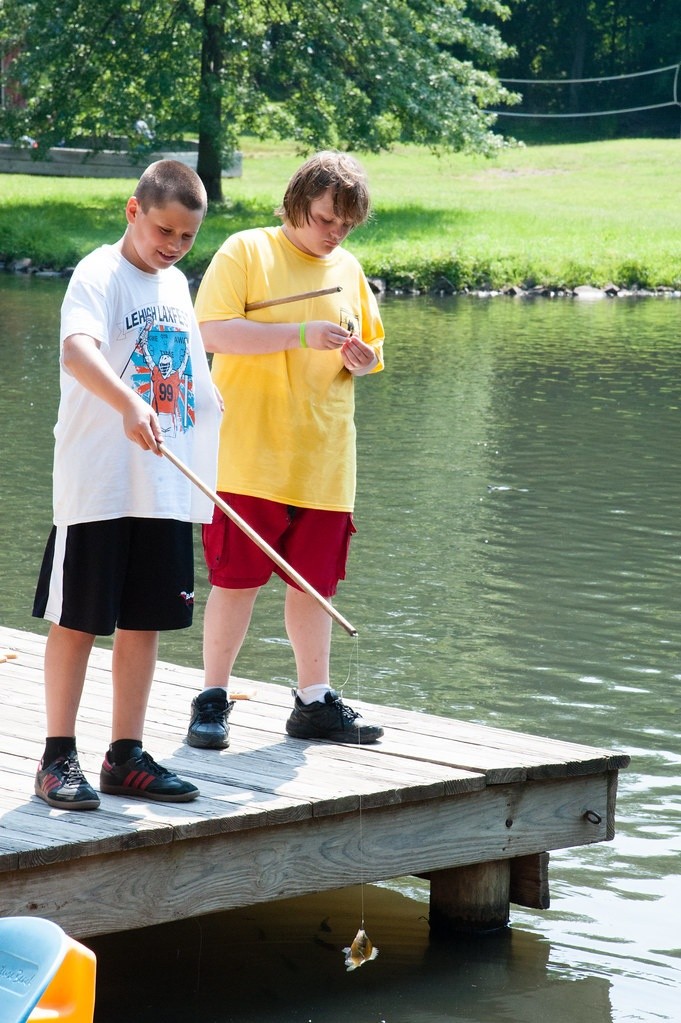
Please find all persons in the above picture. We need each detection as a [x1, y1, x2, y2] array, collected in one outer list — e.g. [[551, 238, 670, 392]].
[[14, 103, 158, 152], [32, 159, 226, 812], [186, 150, 385, 751]]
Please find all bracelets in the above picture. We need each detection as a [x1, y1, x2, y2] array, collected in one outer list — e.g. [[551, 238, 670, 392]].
[[299, 323, 308, 349]]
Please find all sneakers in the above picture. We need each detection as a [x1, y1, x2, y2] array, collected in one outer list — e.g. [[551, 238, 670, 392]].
[[187, 688, 236, 748], [99, 752, 200, 802], [34, 749, 103, 810], [286, 685, 384, 741]]
